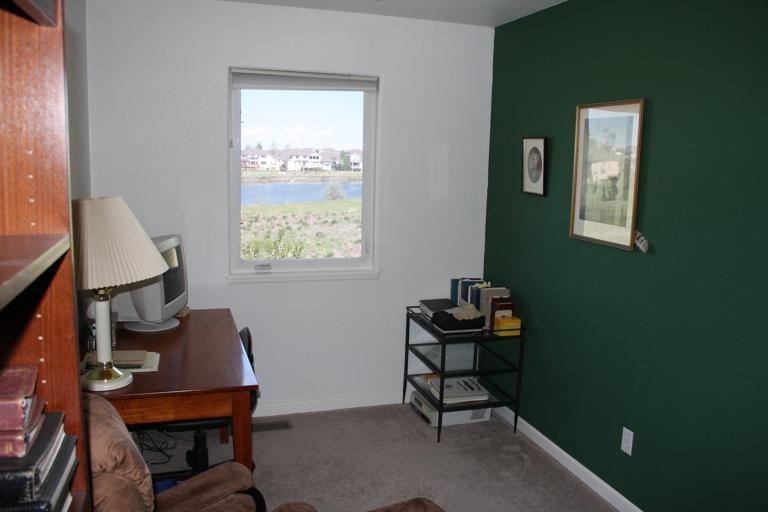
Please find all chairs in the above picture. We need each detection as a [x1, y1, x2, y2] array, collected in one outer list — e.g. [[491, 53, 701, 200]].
[[81, 393, 441, 512], [125, 327, 258, 466]]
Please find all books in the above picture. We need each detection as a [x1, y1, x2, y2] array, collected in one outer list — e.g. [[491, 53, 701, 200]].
[[1, 366, 80, 512], [450, 276, 513, 329], [419, 298, 457, 321], [429, 376, 490, 404], [81, 349, 160, 372]]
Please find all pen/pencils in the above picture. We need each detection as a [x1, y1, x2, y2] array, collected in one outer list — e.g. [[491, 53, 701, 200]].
[[85, 365, 142, 370]]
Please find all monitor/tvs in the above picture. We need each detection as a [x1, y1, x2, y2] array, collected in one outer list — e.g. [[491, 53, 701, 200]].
[[86, 234, 189, 332]]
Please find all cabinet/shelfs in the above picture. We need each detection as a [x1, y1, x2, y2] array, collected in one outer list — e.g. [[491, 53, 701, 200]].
[[402, 312, 525, 442], [0, 0, 94, 512]]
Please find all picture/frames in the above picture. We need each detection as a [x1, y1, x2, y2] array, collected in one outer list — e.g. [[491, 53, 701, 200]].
[[569, 97, 645, 252], [521, 137, 547, 197]]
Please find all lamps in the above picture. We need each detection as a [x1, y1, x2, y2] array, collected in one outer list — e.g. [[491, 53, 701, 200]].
[[72, 196, 169, 391]]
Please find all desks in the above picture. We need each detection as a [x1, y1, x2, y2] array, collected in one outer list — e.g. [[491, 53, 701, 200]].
[[82, 307, 259, 472]]
